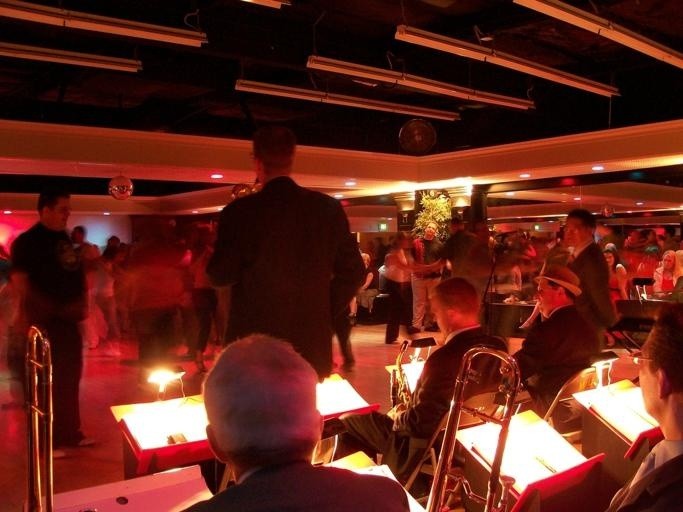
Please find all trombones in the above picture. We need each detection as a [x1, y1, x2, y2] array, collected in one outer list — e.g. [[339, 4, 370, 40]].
[[426, 348, 520, 511], [25, 326, 53, 510]]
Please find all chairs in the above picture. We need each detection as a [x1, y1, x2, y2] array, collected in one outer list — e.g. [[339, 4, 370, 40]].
[[543, 366, 612, 444], [403, 386, 506, 506]]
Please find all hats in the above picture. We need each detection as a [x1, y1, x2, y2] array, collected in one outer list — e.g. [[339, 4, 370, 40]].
[[534, 265, 582, 296]]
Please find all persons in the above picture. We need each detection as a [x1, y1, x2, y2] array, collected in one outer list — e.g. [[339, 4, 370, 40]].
[[9, 188, 95, 448], [605, 304, 682, 512], [336, 216, 484, 372], [0, 256, 26, 371], [511, 264, 599, 403], [340, 278, 508, 467], [207, 123, 363, 392], [70, 222, 227, 362], [174, 332, 411, 512], [494, 209, 682, 350]]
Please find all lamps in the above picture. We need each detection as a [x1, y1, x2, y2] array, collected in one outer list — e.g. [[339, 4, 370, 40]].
[[631, 277, 655, 305], [406, 336, 436, 363], [589, 350, 618, 389], [146, 364, 187, 402]]
